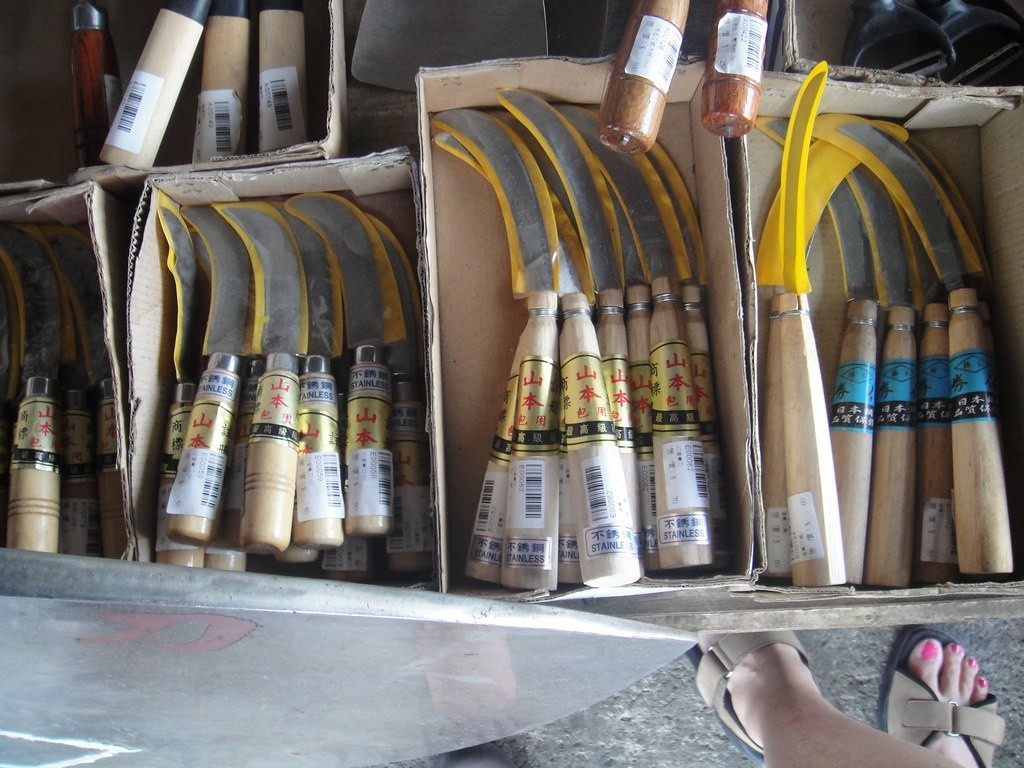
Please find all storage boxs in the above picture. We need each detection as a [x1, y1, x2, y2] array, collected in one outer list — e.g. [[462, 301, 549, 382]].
[[0, 0, 1024, 599]]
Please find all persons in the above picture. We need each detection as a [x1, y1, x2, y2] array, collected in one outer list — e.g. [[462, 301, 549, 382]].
[[685, 625, 1006, 767]]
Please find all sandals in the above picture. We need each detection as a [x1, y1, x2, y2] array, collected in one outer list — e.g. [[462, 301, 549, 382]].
[[877, 624, 1006, 768], [685, 628, 821, 768]]
